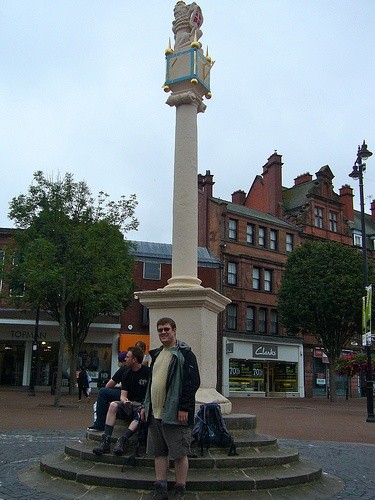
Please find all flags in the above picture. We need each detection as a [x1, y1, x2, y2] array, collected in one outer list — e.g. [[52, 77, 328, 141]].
[[362, 288, 372, 331]]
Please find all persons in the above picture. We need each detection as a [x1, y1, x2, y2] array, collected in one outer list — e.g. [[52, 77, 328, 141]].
[[135, 341, 149, 366], [76, 366, 92, 403], [87, 351, 128, 432], [141, 318, 200, 500], [93, 347, 150, 455]]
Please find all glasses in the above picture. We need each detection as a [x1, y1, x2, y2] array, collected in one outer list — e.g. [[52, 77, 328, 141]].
[[158, 328, 171, 333]]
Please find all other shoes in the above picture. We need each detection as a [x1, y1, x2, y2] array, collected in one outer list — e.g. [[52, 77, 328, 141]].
[[172, 483, 186, 500], [151, 482, 168, 500], [113, 435, 128, 455], [93, 441, 110, 454]]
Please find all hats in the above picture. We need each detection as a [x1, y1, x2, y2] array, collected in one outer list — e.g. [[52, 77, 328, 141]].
[[118, 352, 127, 362]]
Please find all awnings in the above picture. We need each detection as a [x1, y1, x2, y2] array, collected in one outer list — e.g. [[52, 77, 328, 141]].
[[315, 351, 373, 364]]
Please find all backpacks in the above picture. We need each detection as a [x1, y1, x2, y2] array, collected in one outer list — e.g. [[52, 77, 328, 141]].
[[194, 403, 232, 449]]
